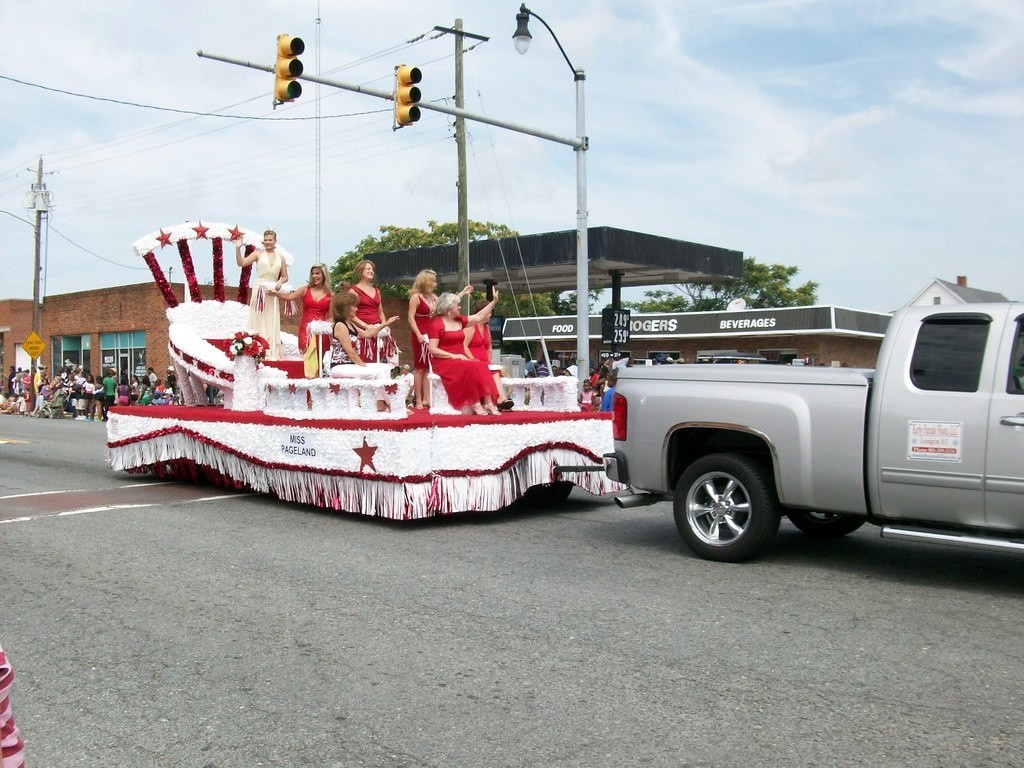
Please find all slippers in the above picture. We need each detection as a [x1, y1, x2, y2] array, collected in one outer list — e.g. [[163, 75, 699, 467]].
[[497, 398, 514, 409]]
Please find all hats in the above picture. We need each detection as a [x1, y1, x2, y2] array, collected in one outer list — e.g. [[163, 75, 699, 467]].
[[399, 364, 409, 370], [601, 366, 608, 371]]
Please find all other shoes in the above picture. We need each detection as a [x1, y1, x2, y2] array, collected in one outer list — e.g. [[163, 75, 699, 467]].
[[415, 404, 423, 409], [384, 408, 391, 413], [71, 414, 108, 423], [406, 408, 414, 416], [422, 401, 430, 407]]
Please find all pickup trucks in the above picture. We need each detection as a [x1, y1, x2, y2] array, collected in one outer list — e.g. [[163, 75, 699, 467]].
[[597, 301, 1023, 566]]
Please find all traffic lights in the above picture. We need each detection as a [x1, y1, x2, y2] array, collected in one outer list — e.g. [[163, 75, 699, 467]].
[[394, 63, 422, 131], [273, 32, 306, 110]]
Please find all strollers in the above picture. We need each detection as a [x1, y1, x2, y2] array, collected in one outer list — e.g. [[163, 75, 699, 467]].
[[35, 389, 66, 419]]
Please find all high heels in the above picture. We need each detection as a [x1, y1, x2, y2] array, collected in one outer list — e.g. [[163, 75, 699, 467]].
[[484, 403, 502, 416], [471, 403, 488, 416]]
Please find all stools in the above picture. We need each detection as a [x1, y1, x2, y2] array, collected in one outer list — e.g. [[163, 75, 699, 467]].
[[427, 374, 462, 417]]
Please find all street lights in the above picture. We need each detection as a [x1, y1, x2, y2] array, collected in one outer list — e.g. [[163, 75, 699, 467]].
[[513, 3, 589, 408]]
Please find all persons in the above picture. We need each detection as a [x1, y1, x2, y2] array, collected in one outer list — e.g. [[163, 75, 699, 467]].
[[524, 352, 620, 412], [329, 291, 401, 370], [235, 227, 288, 362], [266, 263, 334, 376], [349, 259, 391, 364], [393, 269, 514, 416], [0, 363, 185, 422]]
